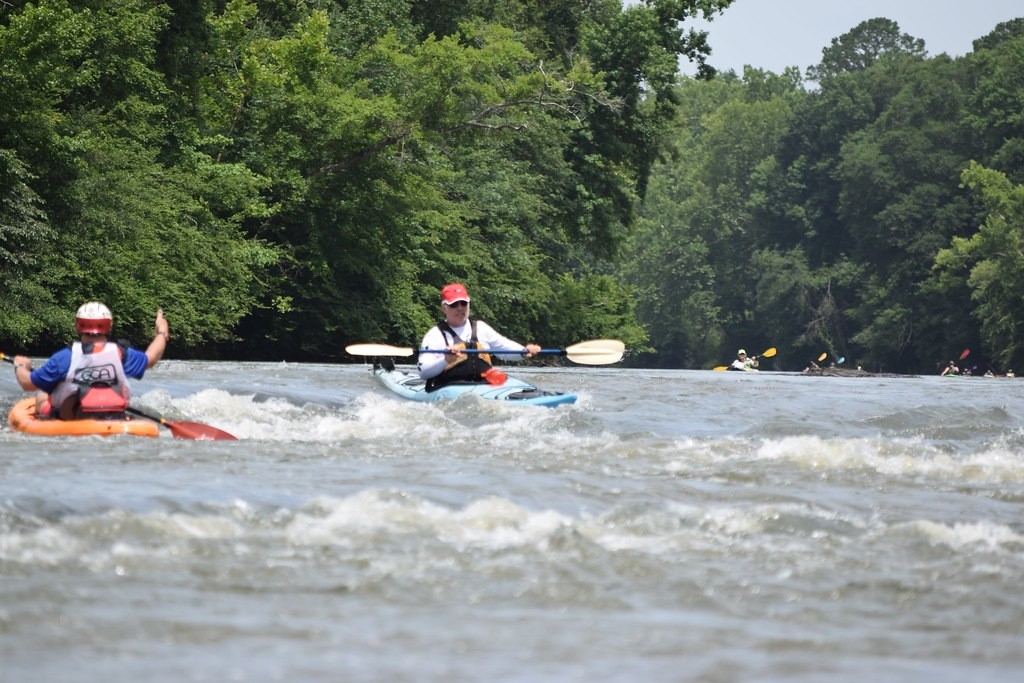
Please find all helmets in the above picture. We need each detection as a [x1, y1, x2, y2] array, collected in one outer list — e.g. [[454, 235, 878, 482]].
[[76, 302, 114, 334]]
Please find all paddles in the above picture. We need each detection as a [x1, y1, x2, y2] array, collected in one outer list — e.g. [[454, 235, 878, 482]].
[[835, 357, 845, 365], [944, 349, 971, 375], [0, 353, 240, 442], [345, 338, 626, 366], [713, 347, 777, 371], [803, 352, 827, 372]]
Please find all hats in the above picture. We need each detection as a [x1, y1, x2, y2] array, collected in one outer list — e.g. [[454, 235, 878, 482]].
[[949, 361, 954, 365], [442, 284, 469, 304], [738, 349, 746, 355]]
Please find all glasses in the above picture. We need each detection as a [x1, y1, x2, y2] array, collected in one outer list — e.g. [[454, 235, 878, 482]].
[[446, 301, 467, 308]]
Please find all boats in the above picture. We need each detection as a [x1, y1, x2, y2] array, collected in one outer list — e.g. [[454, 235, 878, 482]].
[[945, 374, 959, 376], [6, 396, 160, 437], [714, 366, 759, 371], [371, 356, 579, 407], [984, 375, 994, 378]]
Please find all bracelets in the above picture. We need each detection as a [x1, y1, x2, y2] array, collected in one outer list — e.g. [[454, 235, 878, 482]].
[[155, 330, 170, 341], [14, 363, 27, 373]]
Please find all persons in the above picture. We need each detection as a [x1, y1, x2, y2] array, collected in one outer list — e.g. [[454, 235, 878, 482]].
[[941, 361, 960, 376], [962, 368, 1013, 378], [13, 301, 170, 420], [732, 349, 759, 370], [808, 362, 820, 370], [829, 362, 837, 369], [419, 282, 541, 391]]
